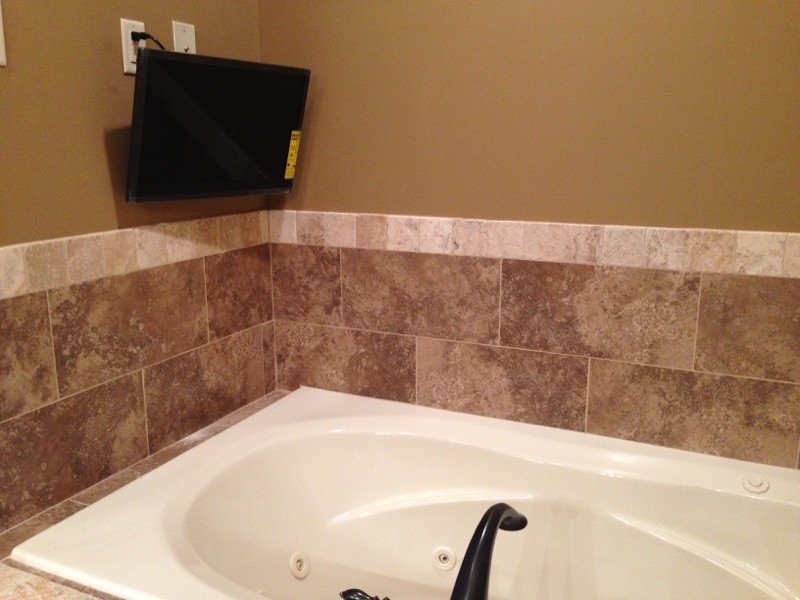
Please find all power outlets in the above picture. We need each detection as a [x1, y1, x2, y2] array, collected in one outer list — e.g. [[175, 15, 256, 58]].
[[120, 18, 147, 75]]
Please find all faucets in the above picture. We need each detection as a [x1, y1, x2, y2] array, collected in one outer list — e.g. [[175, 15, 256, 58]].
[[441, 502, 529, 600]]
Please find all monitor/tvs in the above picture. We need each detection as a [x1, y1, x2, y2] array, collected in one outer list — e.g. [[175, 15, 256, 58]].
[[125, 45, 313, 203]]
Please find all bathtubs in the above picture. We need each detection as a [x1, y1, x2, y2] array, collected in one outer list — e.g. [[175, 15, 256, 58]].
[[0, 366, 800, 600]]
